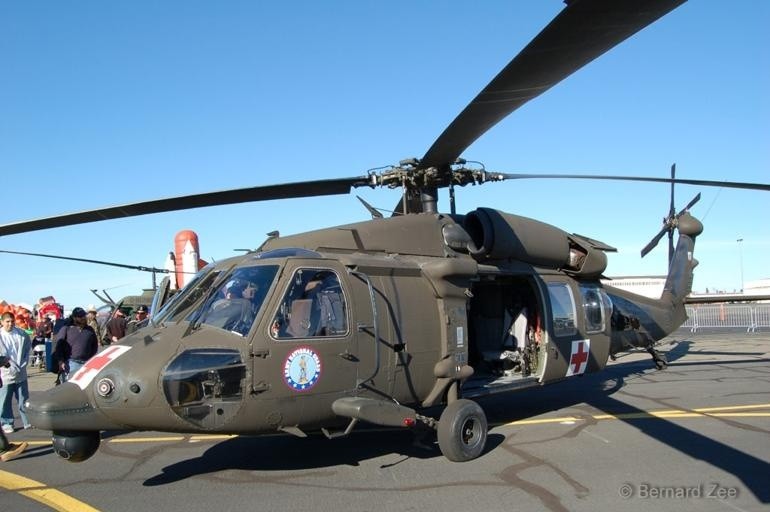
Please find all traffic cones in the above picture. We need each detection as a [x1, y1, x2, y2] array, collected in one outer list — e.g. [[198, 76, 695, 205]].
[[718, 303, 727, 322]]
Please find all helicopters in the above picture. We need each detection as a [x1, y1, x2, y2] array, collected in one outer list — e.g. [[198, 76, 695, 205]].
[[0, 249, 196, 353], [1, 0, 770, 467]]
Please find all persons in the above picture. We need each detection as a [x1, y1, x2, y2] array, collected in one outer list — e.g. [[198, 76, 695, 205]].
[[0, 305, 149, 462]]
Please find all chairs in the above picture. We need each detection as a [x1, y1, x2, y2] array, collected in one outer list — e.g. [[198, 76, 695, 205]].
[[486, 302, 530, 376], [29, 344, 46, 368]]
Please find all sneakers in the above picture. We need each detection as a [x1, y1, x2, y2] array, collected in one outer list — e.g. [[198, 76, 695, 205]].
[[2, 423, 15, 434], [0, 441, 30, 463]]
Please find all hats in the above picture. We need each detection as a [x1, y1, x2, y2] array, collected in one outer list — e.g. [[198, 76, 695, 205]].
[[118, 304, 148, 315], [72, 307, 88, 317]]
